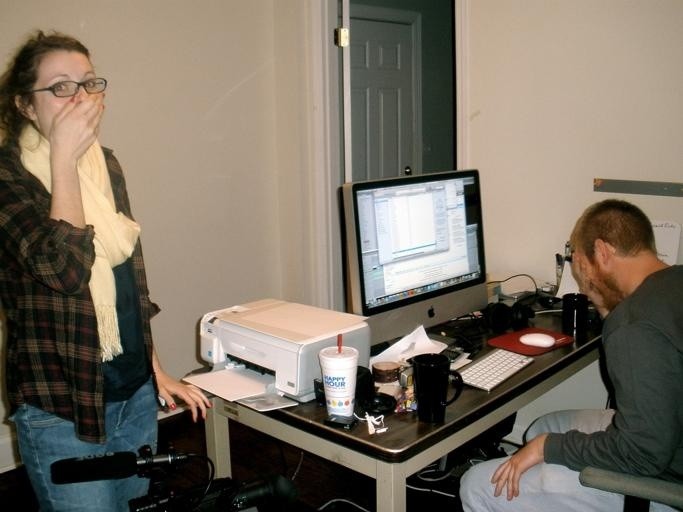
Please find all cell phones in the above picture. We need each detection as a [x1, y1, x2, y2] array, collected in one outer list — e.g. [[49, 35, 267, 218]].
[[439, 345, 463, 363]]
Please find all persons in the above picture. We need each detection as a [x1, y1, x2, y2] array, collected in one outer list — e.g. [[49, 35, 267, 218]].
[[0, 27, 213, 512], [456, 199, 683, 512]]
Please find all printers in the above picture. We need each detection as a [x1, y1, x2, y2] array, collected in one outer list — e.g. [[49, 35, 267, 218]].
[[200, 299, 371, 412]]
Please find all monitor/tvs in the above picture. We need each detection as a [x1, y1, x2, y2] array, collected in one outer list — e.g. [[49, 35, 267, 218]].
[[336, 168, 489, 353]]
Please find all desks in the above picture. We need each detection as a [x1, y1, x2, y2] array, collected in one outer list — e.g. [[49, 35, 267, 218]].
[[181, 293, 609, 512]]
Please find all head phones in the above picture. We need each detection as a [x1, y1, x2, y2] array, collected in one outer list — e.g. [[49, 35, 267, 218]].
[[479, 300, 536, 335]]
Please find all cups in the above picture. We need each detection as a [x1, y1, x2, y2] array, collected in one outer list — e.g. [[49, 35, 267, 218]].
[[411, 352, 463, 425], [562, 293, 589, 334], [318, 345, 359, 418]]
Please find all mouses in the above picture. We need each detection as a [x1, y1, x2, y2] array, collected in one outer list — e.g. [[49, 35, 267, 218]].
[[519, 332, 555, 348]]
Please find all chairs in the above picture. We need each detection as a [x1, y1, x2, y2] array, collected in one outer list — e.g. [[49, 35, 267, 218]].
[[578, 466, 683, 512]]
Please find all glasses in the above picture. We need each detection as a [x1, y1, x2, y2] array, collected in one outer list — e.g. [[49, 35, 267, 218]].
[[22, 78, 107, 98]]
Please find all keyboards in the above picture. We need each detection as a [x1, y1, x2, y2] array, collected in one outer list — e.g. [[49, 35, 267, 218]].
[[449, 348, 535, 392]]
